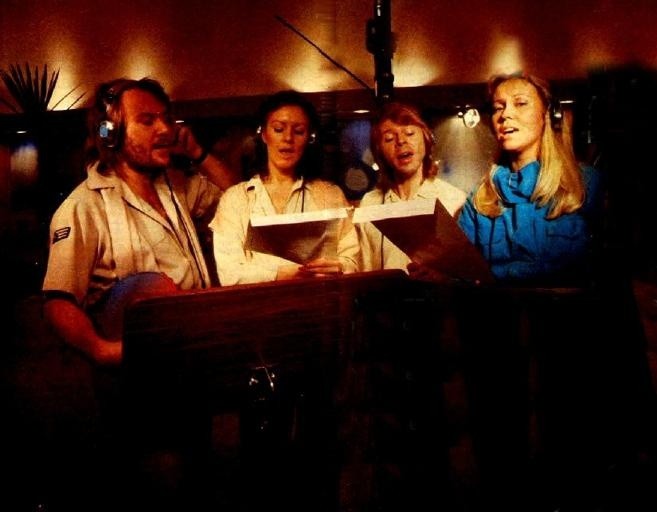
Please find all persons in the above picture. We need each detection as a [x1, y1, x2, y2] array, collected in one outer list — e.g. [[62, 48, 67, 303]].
[[39, 79, 243, 434], [406, 72, 599, 290], [354, 103, 476, 276], [205, 89, 355, 290]]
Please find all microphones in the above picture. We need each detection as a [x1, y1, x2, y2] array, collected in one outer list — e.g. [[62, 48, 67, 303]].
[[365, 0, 396, 104]]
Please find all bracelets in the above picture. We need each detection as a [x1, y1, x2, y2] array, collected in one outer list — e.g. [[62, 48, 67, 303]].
[[190, 151, 210, 171]]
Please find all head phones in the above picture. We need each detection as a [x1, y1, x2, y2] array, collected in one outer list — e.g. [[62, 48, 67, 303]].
[[368, 108, 436, 177], [255, 93, 325, 147], [96, 83, 179, 150], [546, 97, 564, 129]]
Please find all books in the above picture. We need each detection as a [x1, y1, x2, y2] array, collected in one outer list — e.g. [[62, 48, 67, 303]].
[[246, 208, 347, 266], [354, 200, 491, 281]]
